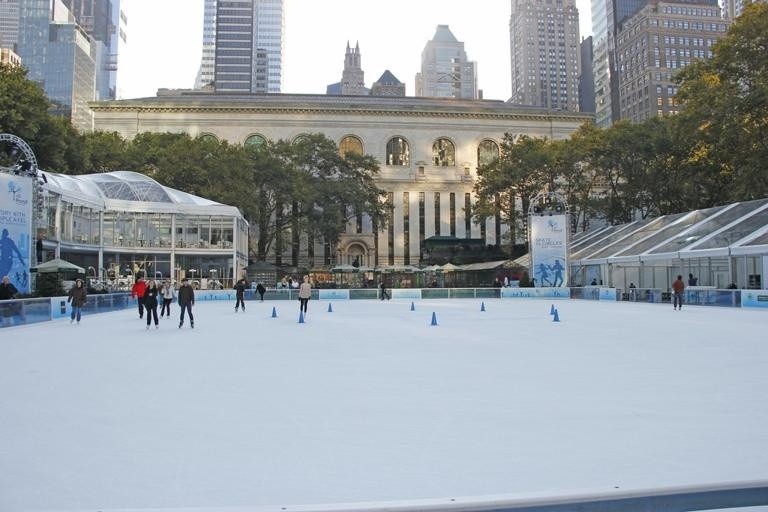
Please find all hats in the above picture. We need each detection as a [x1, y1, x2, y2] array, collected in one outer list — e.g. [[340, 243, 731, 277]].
[[181, 278, 188, 281]]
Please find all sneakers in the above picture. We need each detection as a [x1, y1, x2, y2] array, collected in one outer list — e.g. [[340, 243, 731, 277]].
[[190, 320, 194, 327], [179, 320, 184, 326]]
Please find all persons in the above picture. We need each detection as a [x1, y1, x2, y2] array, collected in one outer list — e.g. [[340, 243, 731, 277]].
[[688, 273, 698, 285], [254, 282, 267, 301], [281, 275, 288, 289], [1, 274, 26, 318], [66, 279, 88, 324], [158, 282, 165, 308], [142, 280, 160, 327], [13, 270, 22, 286], [362, 272, 369, 289], [629, 282, 638, 296], [20, 270, 30, 287], [298, 275, 311, 318], [160, 280, 177, 318], [548, 259, 565, 286], [0, 228, 27, 281], [379, 281, 391, 301], [289, 275, 293, 289], [132, 275, 148, 319], [234, 279, 247, 312], [178, 277, 195, 328], [534, 263, 553, 287], [493, 277, 502, 298], [673, 275, 685, 311]]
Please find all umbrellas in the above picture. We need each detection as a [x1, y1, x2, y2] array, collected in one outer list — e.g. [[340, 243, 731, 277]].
[[30, 255, 85, 297]]
